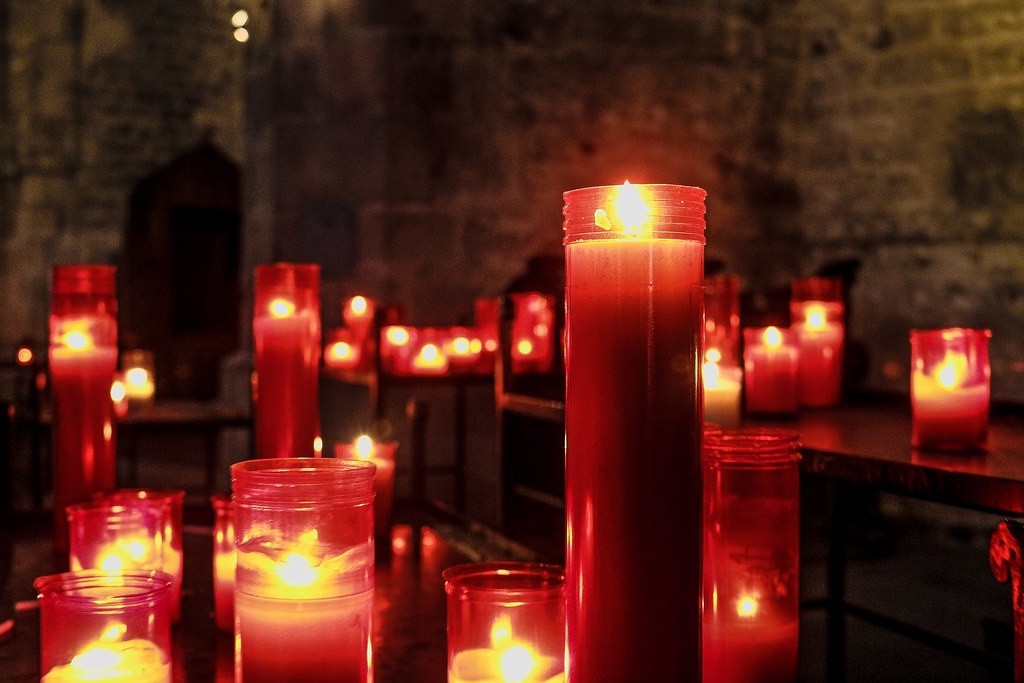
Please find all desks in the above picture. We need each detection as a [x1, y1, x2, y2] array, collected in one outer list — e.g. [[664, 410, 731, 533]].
[[326, 367, 493, 529], [745, 400, 1024, 682], [494, 387, 569, 566], [121, 399, 252, 517]]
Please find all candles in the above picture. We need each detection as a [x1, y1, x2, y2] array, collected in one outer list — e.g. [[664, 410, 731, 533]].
[[35, 177, 989, 683]]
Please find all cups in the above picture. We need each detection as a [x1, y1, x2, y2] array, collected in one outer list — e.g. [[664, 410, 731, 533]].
[[212, 494, 235, 633], [705, 276, 742, 429], [112, 488, 183, 623], [66, 502, 163, 573], [231, 457, 377, 683], [743, 328, 796, 413], [911, 329, 990, 450], [327, 297, 500, 374], [336, 443, 395, 537], [34, 569, 174, 683], [703, 428, 801, 683], [442, 562, 566, 683], [123, 351, 156, 402], [790, 278, 844, 406], [510, 293, 554, 375]]
